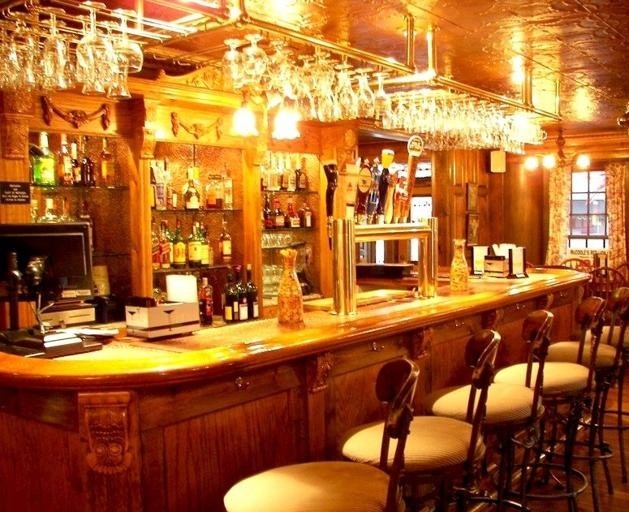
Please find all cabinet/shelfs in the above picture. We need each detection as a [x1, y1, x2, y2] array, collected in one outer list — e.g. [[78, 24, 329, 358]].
[[151, 207, 242, 274], [28, 183, 132, 323], [262, 189, 321, 306]]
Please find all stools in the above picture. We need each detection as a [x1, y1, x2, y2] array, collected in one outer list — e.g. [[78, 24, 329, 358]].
[[585, 268, 626, 298], [423, 308, 554, 510], [495, 294, 608, 512], [585, 325, 627, 485], [538, 286, 627, 496], [563, 258, 591, 275], [341, 329, 502, 512], [222, 359, 421, 512]]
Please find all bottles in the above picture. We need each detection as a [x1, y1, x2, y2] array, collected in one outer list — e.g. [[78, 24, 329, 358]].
[[449, 239, 470, 291], [277, 249, 304, 324], [31, 193, 97, 255], [150, 160, 234, 211], [29, 130, 116, 187], [218, 221, 233, 265], [198, 277, 214, 326], [222, 263, 260, 324], [151, 215, 214, 270], [262, 192, 313, 228], [261, 156, 310, 192]]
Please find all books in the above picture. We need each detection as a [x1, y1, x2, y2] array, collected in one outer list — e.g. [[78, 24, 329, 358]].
[[0, 325, 102, 359]]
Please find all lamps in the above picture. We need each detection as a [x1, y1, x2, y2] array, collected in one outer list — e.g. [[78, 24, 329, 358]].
[[524, 130, 591, 171], [230, 91, 260, 137]]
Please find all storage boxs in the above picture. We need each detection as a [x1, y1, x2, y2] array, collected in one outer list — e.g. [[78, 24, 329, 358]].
[[125, 300, 200, 340]]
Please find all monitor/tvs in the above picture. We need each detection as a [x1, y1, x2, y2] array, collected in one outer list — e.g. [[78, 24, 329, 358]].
[[0, 221, 95, 309]]
